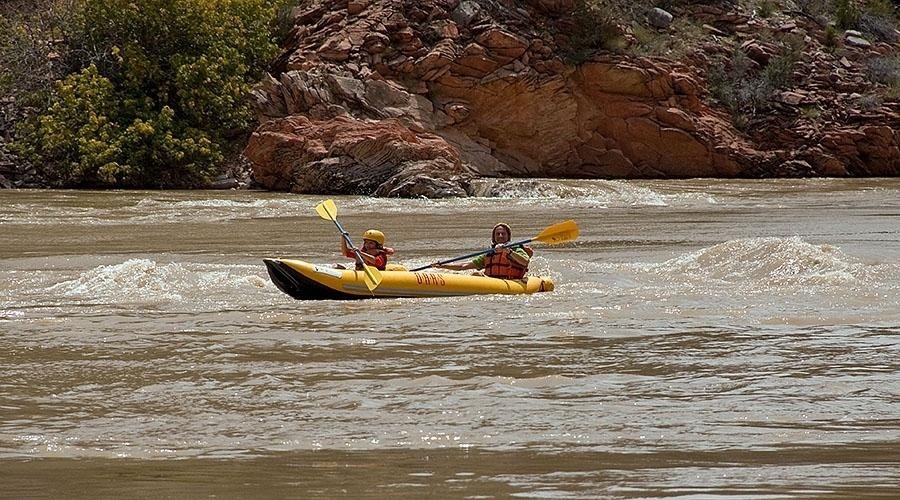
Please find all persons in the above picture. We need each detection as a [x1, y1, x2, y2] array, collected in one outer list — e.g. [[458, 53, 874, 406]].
[[340, 229, 389, 272], [430, 222, 534, 280]]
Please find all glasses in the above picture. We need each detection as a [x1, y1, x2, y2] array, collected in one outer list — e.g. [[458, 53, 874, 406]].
[[362, 229, 385, 245]]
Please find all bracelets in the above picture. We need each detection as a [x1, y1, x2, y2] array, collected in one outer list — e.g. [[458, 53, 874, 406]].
[[508, 249, 513, 256]]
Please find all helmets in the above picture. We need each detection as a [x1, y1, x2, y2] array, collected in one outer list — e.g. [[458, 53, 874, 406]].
[[491, 222, 512, 244]]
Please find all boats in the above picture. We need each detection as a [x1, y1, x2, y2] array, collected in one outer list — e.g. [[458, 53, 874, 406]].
[[263, 257, 554, 300]]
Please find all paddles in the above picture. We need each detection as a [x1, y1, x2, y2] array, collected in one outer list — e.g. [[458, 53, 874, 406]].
[[385, 220, 580, 272], [316, 199, 383, 291]]
